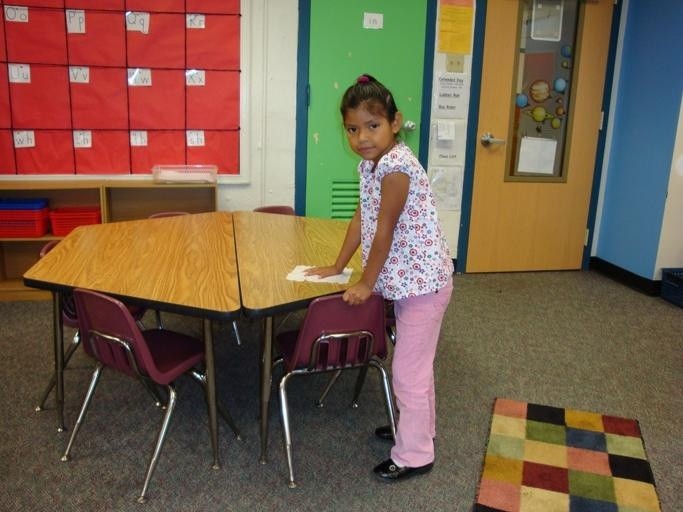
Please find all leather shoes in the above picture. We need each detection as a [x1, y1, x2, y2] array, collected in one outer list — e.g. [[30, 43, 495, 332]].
[[372, 457, 433, 482], [374, 426, 395, 441]]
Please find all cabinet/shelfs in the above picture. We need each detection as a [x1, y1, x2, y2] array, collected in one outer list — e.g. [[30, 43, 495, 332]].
[[0, 180, 217, 302]]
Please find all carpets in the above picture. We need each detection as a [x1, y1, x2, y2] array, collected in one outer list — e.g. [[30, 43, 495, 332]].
[[471, 396, 664, 511]]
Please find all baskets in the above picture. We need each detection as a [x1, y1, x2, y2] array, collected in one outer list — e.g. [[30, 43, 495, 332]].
[[0, 198, 100, 238]]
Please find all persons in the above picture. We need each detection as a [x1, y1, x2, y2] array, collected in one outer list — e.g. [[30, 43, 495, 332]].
[[301, 72, 457, 482]]
